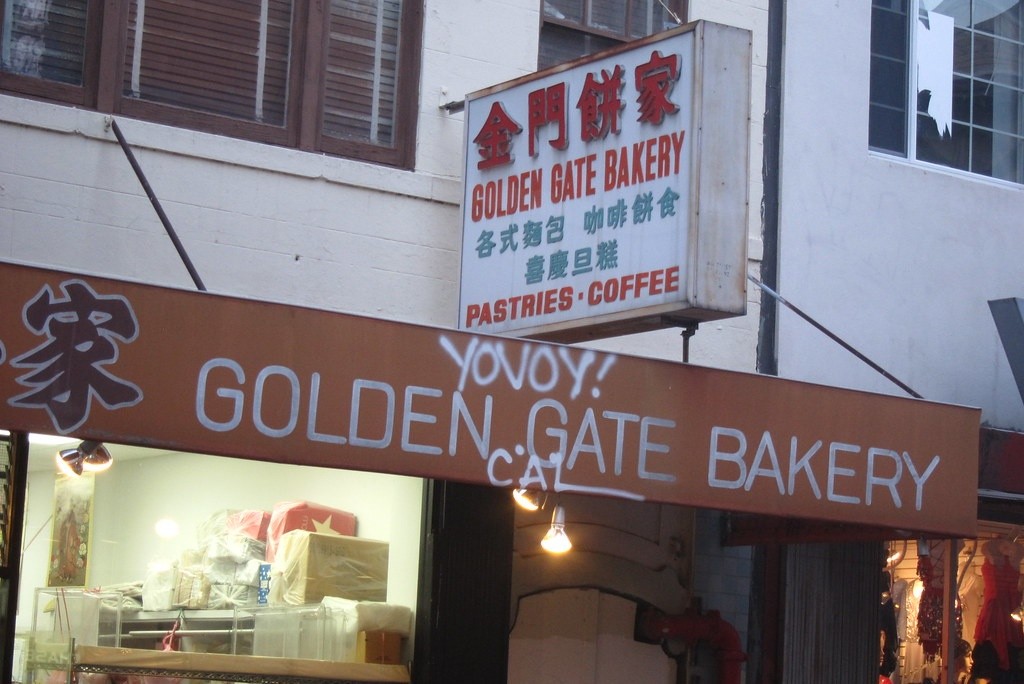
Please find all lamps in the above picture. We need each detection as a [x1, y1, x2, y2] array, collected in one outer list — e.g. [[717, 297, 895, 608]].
[[540, 505, 572, 555], [56, 440, 104, 478], [83, 445, 114, 472], [1010, 608, 1022, 622], [513, 489, 546, 511], [887, 548, 899, 562]]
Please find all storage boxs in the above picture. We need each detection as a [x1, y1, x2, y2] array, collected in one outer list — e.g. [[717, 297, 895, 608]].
[[272, 533, 389, 604], [142, 536, 272, 613], [227, 511, 272, 543], [265, 501, 356, 563]]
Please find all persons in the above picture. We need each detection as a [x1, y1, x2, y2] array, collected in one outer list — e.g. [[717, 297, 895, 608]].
[[879, 550, 900, 676], [914, 538, 967, 673], [975, 535, 1024, 671], [937, 640, 973, 684]]
